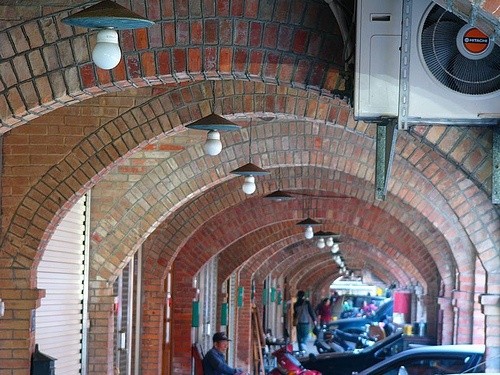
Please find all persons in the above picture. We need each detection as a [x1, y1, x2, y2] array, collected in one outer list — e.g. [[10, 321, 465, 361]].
[[293, 291, 318, 354], [357, 298, 377, 317], [343, 298, 353, 310], [202, 332, 245, 375], [318, 298, 333, 326]]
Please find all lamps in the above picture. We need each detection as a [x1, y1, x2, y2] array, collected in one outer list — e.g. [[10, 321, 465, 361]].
[[262, 167, 296, 202], [229, 126, 271, 195], [325, 231, 338, 246], [314, 226, 328, 248], [295, 204, 322, 239], [185, 81, 242, 156], [331, 240, 342, 253], [59, 0, 156, 71]]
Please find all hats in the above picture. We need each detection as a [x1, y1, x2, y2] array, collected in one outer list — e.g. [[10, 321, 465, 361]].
[[213, 332, 232, 342]]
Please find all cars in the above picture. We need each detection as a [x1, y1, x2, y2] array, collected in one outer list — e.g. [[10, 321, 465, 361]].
[[354, 344, 487, 375], [326, 296, 394, 332], [293, 329, 405, 375]]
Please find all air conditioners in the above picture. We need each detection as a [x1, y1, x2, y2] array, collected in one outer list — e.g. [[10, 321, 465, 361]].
[[352, 0, 500, 124]]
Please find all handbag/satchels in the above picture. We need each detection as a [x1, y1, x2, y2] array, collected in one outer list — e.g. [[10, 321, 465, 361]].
[[293, 318, 299, 326]]
